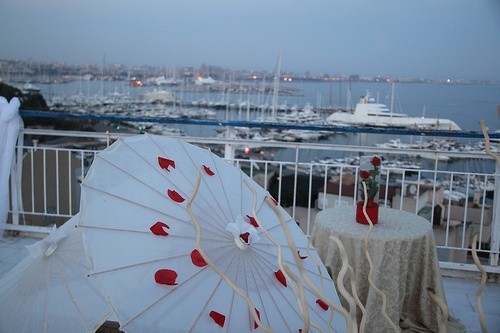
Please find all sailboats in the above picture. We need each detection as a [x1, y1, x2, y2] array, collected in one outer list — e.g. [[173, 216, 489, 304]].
[[0, 38, 499, 206]]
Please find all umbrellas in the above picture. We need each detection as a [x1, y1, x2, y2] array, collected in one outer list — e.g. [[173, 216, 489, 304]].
[[0, 210, 113, 333], [74, 129, 348, 333]]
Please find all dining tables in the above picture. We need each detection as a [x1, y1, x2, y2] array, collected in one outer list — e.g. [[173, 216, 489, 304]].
[[311, 205, 468, 333]]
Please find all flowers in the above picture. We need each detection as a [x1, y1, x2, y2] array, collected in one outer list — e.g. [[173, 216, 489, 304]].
[[359, 156, 381, 207]]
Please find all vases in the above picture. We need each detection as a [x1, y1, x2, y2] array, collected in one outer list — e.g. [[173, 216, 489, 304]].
[[356, 201, 379, 225]]
